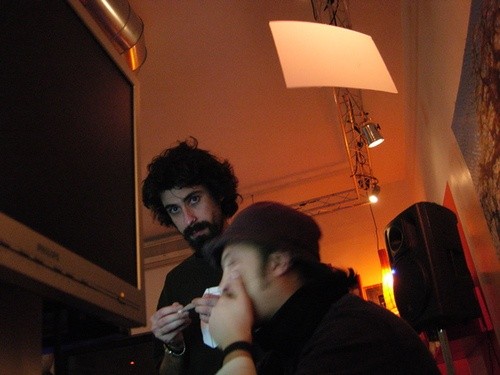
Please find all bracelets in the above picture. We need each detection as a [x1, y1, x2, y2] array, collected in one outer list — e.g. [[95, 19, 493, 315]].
[[221, 340, 252, 357], [163, 342, 185, 358]]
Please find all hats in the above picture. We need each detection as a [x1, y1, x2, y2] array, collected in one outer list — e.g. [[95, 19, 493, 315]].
[[208, 201, 321, 270]]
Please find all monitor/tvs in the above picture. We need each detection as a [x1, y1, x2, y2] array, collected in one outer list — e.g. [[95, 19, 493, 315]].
[[1, 0, 146, 331]]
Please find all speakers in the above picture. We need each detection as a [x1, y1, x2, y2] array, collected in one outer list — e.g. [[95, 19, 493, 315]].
[[385, 202, 481, 332], [54, 333, 164, 375]]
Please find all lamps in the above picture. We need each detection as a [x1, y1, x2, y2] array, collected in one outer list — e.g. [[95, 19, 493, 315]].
[[369, 182, 381, 203], [360, 119, 384, 149]]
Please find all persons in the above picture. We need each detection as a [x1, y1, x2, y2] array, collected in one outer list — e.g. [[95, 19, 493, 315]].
[[141, 142, 443, 375]]
